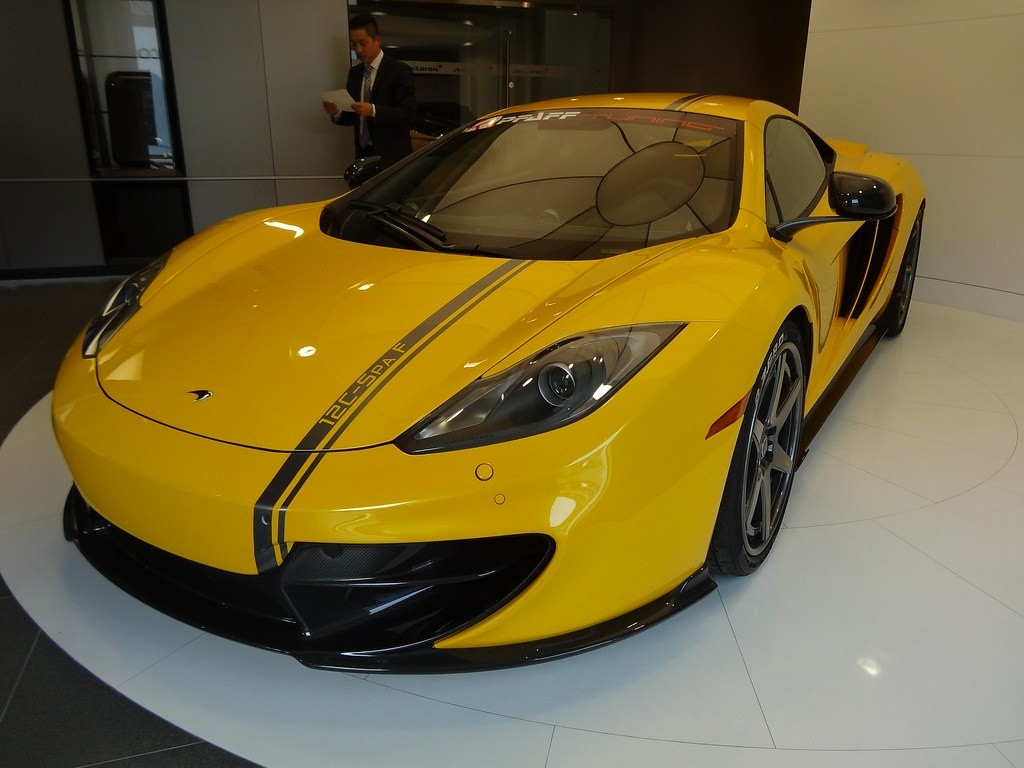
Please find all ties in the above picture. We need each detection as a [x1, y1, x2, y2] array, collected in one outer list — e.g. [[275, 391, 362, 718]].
[[362, 65, 372, 141]]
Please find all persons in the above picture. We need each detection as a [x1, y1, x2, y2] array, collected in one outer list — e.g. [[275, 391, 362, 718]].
[[320, 14, 424, 191]]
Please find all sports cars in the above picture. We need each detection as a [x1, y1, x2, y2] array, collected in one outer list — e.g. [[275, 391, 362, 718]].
[[48, 91, 930, 676]]
